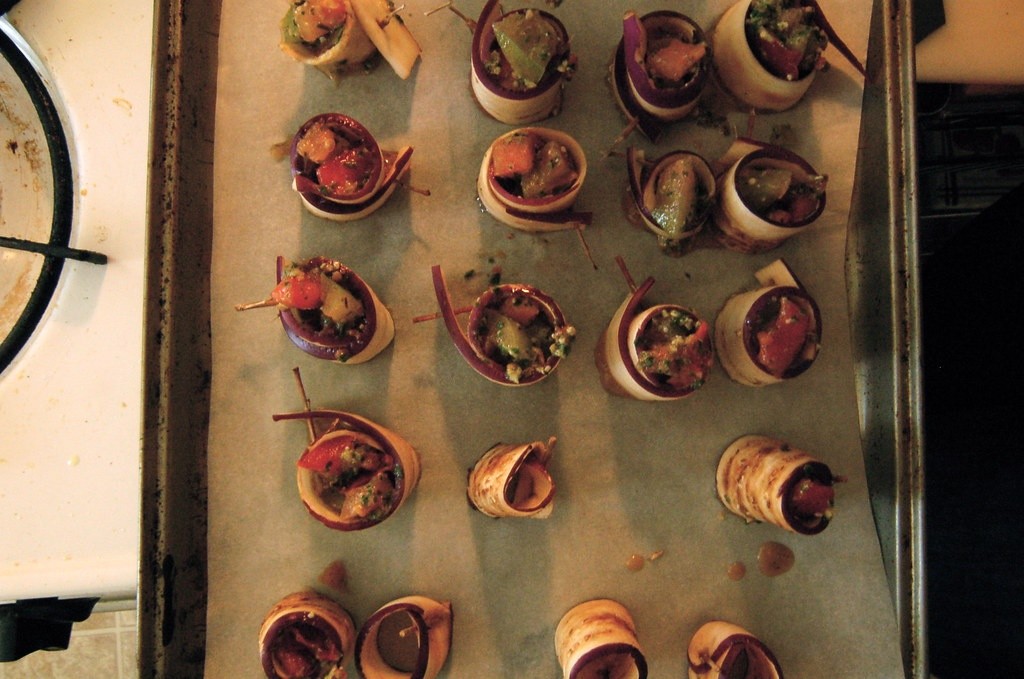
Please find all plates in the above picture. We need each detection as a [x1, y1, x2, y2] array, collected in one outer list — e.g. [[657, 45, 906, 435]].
[[0, 17, 82, 372]]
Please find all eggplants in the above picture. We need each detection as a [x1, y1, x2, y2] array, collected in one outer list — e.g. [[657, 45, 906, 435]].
[[259, 1, 866, 679]]
